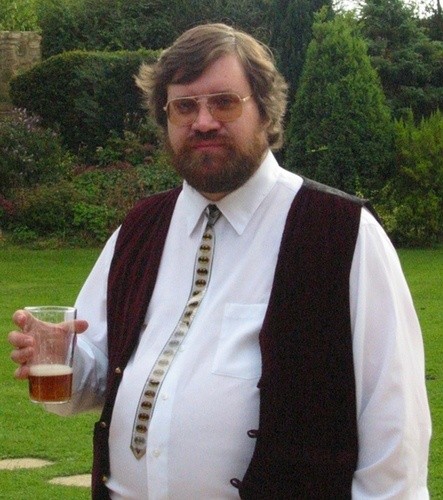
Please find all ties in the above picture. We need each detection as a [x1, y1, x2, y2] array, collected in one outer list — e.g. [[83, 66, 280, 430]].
[[130, 205, 222, 460]]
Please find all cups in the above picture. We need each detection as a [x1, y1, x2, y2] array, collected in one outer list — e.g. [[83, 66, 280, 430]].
[[24, 306, 76, 404]]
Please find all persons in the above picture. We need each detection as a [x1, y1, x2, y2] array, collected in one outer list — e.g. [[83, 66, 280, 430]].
[[9, 23, 433, 500]]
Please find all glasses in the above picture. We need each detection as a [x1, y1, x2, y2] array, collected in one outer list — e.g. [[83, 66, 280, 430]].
[[163, 92, 254, 128]]
